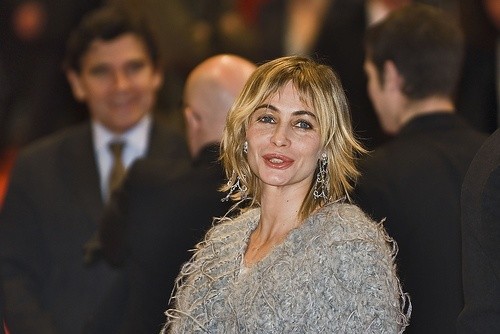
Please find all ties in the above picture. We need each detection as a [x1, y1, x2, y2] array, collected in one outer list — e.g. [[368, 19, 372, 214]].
[[108, 138, 129, 198]]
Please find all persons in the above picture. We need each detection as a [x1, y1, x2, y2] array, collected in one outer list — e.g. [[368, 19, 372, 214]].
[[159, 55, 413, 334], [113, 53, 260, 334], [0, 5, 186, 334], [452, 127, 500, 334], [343, 4, 489, 334]]
